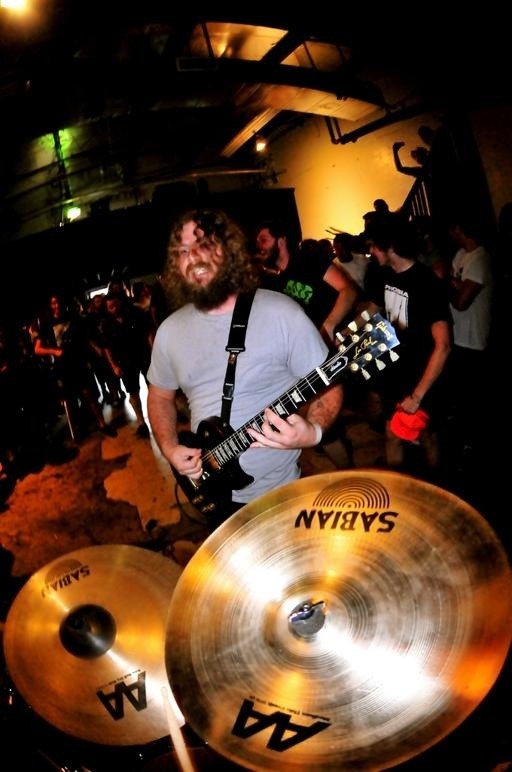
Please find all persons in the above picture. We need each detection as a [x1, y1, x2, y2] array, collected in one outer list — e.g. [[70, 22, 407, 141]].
[[102, 292, 156, 438], [147, 211, 344, 527], [150, 267, 173, 327], [433, 204, 494, 448], [252, 225, 354, 352], [334, 224, 454, 472], [88, 295, 122, 409], [108, 281, 123, 295], [34, 294, 116, 437], [333, 232, 372, 290], [81, 300, 94, 319], [131, 282, 152, 309], [374, 199, 388, 213]]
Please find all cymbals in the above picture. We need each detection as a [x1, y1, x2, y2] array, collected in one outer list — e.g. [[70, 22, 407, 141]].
[[164, 470, 512, 771], [3, 544, 186, 747]]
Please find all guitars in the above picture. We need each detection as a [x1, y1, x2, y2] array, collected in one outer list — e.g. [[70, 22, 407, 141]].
[[167, 283, 402, 521]]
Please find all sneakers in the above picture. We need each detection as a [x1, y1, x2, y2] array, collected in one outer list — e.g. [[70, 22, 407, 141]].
[[52, 447, 80, 466], [110, 414, 127, 428], [136, 424, 150, 439], [100, 424, 117, 438]]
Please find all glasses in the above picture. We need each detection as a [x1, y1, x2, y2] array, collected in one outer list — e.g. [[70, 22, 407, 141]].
[[178, 240, 213, 259]]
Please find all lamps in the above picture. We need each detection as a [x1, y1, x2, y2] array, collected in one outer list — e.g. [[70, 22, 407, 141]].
[[66, 206, 81, 222], [252, 130, 267, 153]]
